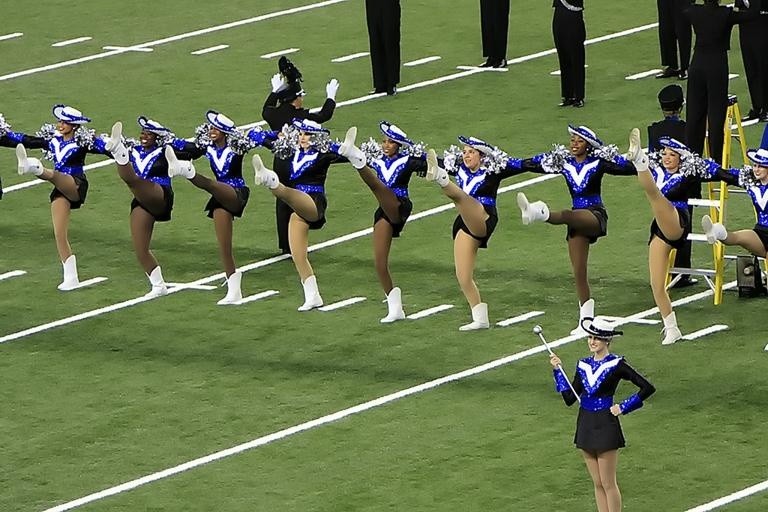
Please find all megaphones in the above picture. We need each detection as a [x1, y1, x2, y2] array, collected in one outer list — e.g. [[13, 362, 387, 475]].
[[736, 256, 762, 296]]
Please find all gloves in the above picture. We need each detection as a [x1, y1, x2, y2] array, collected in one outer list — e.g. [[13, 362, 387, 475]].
[[326, 78, 340, 102], [270, 72, 285, 93]]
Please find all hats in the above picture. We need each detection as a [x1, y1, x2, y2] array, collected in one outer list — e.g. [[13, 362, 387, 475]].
[[378, 120, 415, 147], [746, 147, 768, 167], [278, 81, 307, 104], [656, 134, 693, 159], [658, 84, 686, 112], [52, 103, 91, 124], [458, 135, 495, 155], [581, 314, 624, 340], [135, 115, 176, 136], [567, 124, 604, 148], [205, 108, 238, 134], [291, 117, 331, 137]]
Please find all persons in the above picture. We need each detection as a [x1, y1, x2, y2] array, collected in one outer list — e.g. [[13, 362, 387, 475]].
[[551, 0, 587, 107], [163, 111, 285, 305], [497, 123, 656, 338], [0, 134, 49, 202], [618, 128, 750, 349], [674, 0, 768, 167], [361, 1, 403, 94], [701, 147, 767, 351], [645, 83, 696, 287], [335, 118, 462, 323], [247, 118, 374, 313], [422, 131, 551, 333], [3, 103, 110, 293], [734, 0, 768, 123], [262, 72, 340, 251], [92, 118, 201, 302], [546, 315, 657, 512], [477, 1, 510, 68], [656, 1, 697, 80]]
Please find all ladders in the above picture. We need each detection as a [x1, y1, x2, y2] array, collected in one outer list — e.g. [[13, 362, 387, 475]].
[[663, 93, 768, 305]]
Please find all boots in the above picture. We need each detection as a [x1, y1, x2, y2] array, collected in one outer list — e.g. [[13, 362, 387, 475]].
[[657, 311, 683, 345], [297, 274, 324, 312], [56, 253, 81, 291], [143, 265, 169, 299], [15, 143, 44, 177], [164, 144, 196, 180], [699, 214, 729, 246], [380, 284, 407, 323], [337, 126, 368, 170], [425, 148, 450, 188], [459, 303, 489, 331], [104, 121, 129, 166], [625, 128, 650, 173], [516, 192, 551, 226], [568, 298, 595, 336], [251, 153, 280, 190], [216, 269, 243, 305]]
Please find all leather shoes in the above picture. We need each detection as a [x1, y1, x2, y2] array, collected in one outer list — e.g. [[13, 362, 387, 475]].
[[655, 65, 689, 80], [477, 58, 508, 70], [739, 108, 768, 122], [367, 85, 397, 97], [558, 98, 585, 109]]
